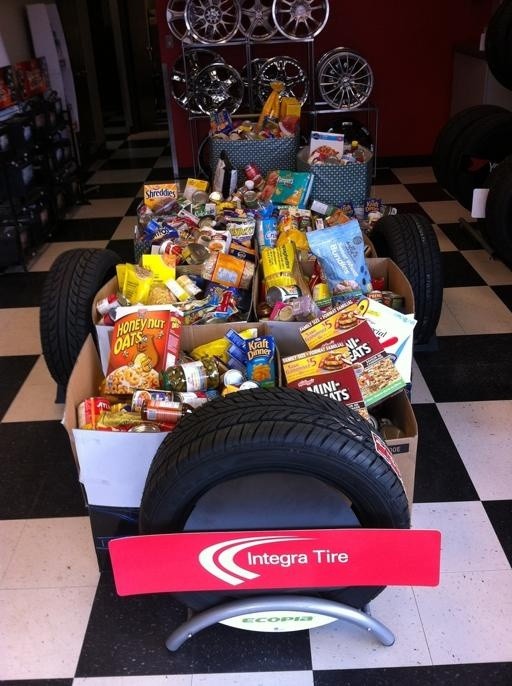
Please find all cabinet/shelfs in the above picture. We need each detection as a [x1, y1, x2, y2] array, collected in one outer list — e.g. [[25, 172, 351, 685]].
[[0, 97, 85, 275]]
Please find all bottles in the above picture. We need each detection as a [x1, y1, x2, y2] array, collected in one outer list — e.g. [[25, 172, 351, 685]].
[[175, 273, 204, 300], [265, 284, 302, 307], [351, 141, 359, 152], [244, 181, 257, 208], [307, 199, 335, 216], [141, 399, 198, 423]]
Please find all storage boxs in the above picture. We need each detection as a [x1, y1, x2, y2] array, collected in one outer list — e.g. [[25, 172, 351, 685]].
[[59, 117, 418, 512]]
